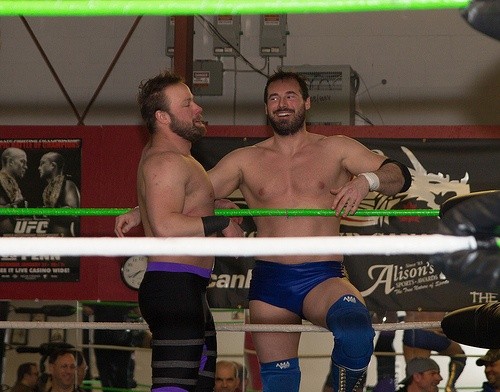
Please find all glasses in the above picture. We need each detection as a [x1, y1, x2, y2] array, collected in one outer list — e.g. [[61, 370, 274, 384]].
[[78, 366, 88, 370], [30, 371, 39, 377]]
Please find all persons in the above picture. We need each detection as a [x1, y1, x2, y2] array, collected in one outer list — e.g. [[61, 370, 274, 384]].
[[402, 305, 467, 391], [398, 356, 443, 392], [476, 347, 500, 392], [33, 151, 80, 237], [115, 70, 412, 391], [214, 360, 240, 392], [48, 342, 78, 392], [5, 363, 52, 392], [138, 74, 248, 391], [0, 147, 30, 233], [74, 350, 87, 392]]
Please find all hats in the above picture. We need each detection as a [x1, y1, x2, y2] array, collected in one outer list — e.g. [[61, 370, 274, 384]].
[[476, 349, 500, 366], [398, 357, 440, 384]]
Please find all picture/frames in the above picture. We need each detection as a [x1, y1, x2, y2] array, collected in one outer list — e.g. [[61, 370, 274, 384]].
[[30, 314, 47, 322], [10, 329, 29, 346], [49, 329, 67, 343]]
